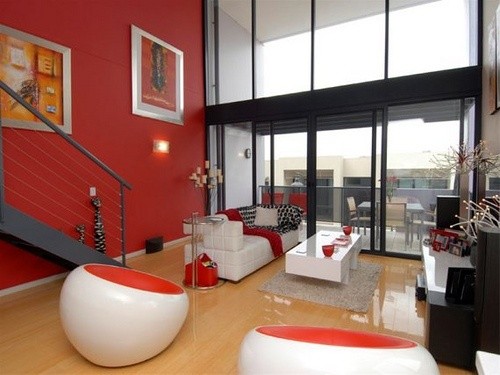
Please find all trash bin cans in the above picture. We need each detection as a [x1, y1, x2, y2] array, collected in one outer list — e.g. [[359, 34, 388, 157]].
[[185, 252, 219, 285]]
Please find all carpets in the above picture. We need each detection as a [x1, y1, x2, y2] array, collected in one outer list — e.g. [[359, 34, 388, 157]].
[[258, 258, 383, 313]]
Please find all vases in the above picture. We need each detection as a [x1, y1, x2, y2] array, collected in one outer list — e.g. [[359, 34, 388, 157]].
[[388, 193, 392, 202]]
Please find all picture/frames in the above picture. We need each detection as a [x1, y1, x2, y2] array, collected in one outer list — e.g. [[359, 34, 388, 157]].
[[0, 25, 72, 135], [131, 24, 184, 125]]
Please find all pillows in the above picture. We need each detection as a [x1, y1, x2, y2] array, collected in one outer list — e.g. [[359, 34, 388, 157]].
[[255, 207, 279, 226]]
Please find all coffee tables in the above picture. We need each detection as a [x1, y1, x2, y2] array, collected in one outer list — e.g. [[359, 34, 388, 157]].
[[284, 230, 362, 284]]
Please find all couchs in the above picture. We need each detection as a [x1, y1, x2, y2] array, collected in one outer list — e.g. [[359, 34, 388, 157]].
[[185, 203, 302, 282]]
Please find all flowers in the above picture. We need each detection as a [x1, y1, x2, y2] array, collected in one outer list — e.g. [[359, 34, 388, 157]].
[[379, 175, 398, 197]]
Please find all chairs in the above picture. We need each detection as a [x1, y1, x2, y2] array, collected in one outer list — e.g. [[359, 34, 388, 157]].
[[376, 195, 409, 251], [58, 263, 190, 367], [240, 324, 440, 375], [409, 204, 437, 248], [288, 193, 307, 216], [262, 193, 284, 207], [346, 196, 371, 236]]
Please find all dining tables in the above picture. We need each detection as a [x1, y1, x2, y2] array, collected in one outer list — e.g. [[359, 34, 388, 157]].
[[358, 200, 425, 251]]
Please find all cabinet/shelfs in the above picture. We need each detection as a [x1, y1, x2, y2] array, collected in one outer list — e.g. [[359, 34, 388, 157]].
[[422, 224, 500, 370]]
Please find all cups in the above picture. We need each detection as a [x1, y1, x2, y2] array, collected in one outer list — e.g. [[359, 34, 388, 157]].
[[343, 226, 351, 235], [322, 245, 334, 257]]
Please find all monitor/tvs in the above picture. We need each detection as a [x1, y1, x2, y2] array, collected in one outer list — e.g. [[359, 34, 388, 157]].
[[437, 196, 461, 230]]
